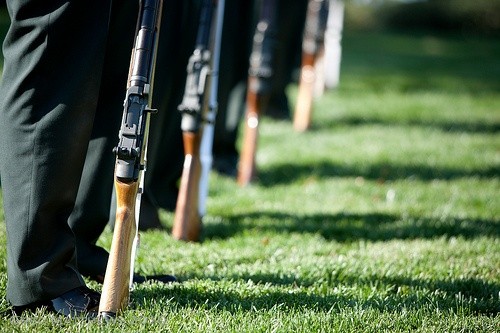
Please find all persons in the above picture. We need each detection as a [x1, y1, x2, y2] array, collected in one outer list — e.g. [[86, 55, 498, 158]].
[[0, 0, 329, 321]]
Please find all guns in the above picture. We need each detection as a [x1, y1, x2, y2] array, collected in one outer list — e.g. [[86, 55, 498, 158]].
[[169, 0, 227, 241], [95, 0, 165, 321], [293, 1, 343, 135], [234, 0, 283, 186]]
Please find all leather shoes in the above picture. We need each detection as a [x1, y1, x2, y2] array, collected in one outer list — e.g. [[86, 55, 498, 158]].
[[88, 261, 178, 284], [7, 287, 100, 318]]
